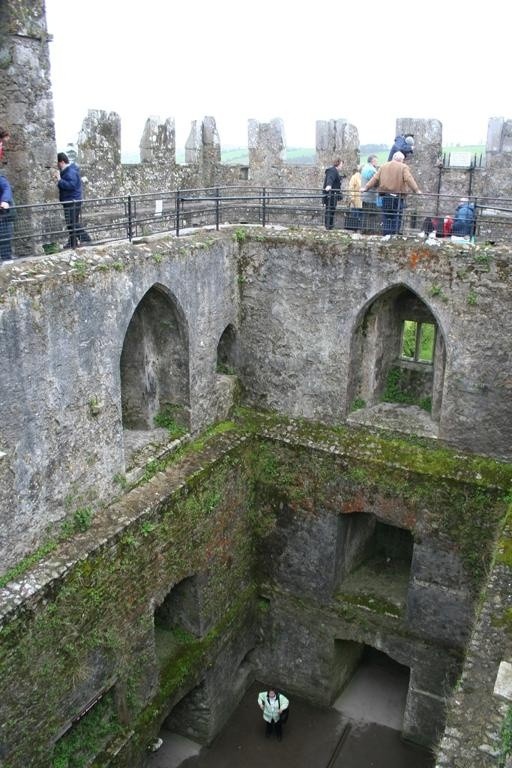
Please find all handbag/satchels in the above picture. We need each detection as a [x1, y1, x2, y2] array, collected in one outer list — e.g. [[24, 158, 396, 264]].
[[280, 708, 291, 724]]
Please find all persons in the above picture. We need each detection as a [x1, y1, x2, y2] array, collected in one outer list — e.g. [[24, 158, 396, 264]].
[[257, 687, 289, 741], [358, 150, 423, 235], [359, 154, 381, 235], [53, 151, 94, 250], [387, 135, 417, 165], [420, 197, 475, 237], [321, 156, 345, 230], [0, 173, 19, 261], [347, 163, 365, 221]]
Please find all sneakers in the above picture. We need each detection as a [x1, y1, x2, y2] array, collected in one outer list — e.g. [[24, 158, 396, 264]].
[[263, 731, 283, 742]]
